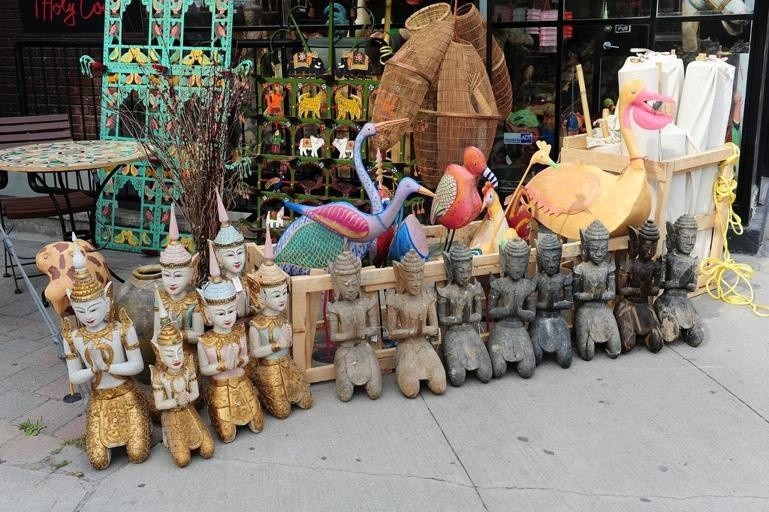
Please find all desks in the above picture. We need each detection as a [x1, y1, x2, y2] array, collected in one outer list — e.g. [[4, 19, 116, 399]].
[[0, 135, 160, 311]]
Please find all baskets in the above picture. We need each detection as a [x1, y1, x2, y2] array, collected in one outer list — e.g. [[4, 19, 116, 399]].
[[365, 2, 520, 203]]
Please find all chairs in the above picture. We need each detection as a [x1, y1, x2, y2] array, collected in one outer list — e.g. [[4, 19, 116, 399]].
[[1, 111, 100, 296]]
